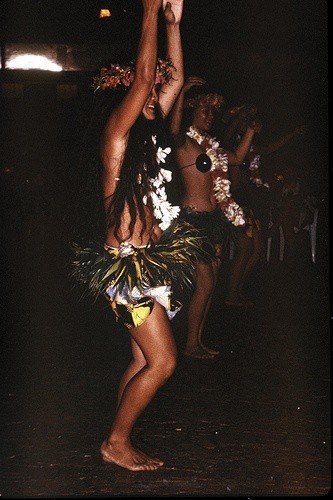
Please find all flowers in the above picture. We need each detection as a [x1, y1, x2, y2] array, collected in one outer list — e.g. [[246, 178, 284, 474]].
[[93, 56, 173, 90], [139, 135, 181, 232], [249, 144, 271, 190], [182, 92, 225, 110], [186, 126, 249, 228]]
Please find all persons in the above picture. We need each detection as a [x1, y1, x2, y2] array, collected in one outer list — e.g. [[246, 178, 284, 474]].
[[215, 102, 311, 307], [167, 77, 266, 360], [73, 0, 218, 472]]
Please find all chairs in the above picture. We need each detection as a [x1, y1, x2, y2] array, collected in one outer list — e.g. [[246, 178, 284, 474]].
[[266, 207, 321, 263]]
